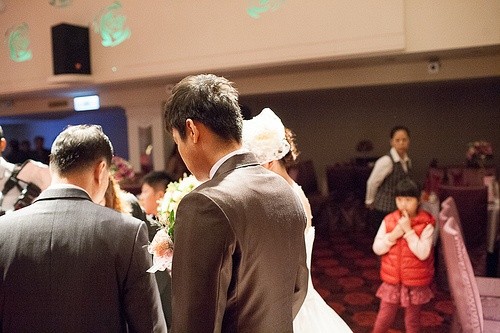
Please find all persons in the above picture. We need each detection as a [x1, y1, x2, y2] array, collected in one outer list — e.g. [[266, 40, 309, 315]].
[[0, 125, 16, 200], [234, 105, 346, 333], [0, 121, 168, 333], [2, 131, 50, 171], [361, 124, 418, 276], [139, 169, 177, 230], [160, 70, 307, 333], [168, 141, 188, 181], [368, 177, 437, 332]]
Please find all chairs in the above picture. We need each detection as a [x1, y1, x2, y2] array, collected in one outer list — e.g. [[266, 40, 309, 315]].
[[438, 185, 500, 333]]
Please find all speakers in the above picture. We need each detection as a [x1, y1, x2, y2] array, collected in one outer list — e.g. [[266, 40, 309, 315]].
[[53, 23, 91, 75]]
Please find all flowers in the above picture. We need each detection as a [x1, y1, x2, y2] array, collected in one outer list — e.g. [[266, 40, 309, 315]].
[[142, 173, 200, 279], [467, 141, 493, 159]]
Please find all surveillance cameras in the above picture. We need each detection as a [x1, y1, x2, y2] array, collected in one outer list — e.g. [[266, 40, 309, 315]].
[[428, 58, 442, 73]]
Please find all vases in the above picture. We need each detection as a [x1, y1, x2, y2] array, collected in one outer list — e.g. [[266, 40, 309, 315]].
[[477, 160, 487, 168]]
